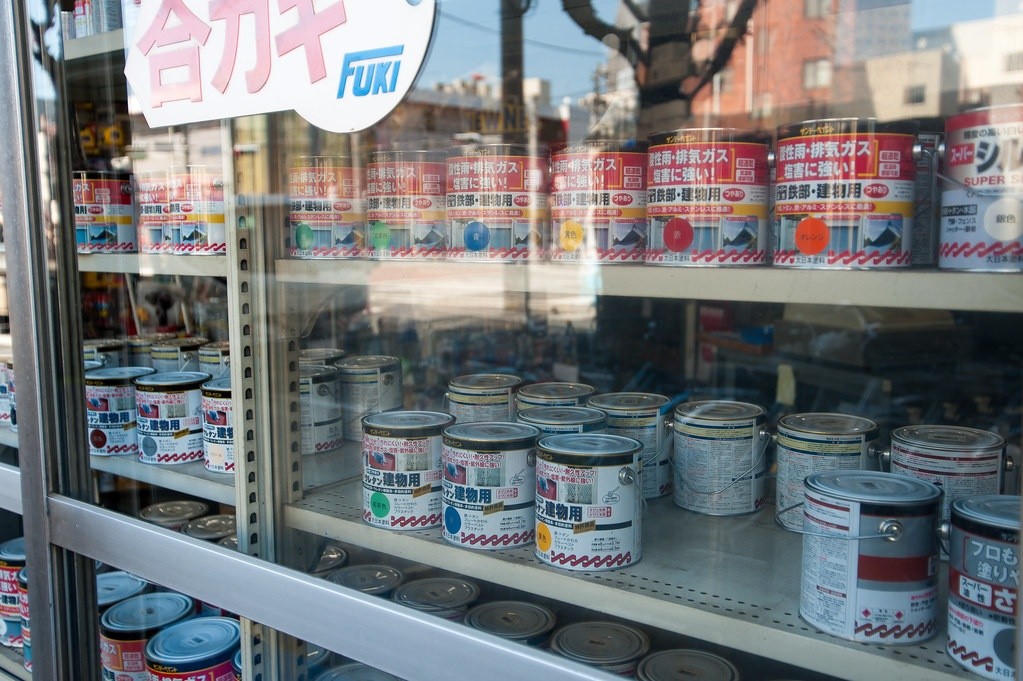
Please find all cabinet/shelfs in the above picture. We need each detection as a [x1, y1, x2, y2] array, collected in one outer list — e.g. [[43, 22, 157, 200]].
[[0, 0, 1023, 681]]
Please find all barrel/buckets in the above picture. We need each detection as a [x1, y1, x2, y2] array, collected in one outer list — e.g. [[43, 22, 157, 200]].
[[70, 102, 1023, 274], [1, 328, 1022, 681]]
